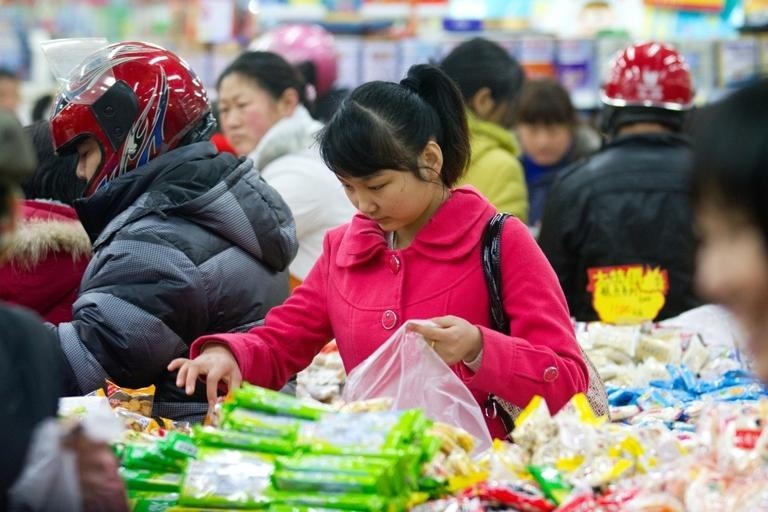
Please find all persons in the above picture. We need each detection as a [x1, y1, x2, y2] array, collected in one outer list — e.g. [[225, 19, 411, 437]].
[[169, 64, 587, 439], [433, 34, 766, 385], [0, 21, 357, 512]]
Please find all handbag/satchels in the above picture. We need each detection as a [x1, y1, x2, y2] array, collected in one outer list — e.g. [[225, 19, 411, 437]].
[[477, 209, 616, 446]]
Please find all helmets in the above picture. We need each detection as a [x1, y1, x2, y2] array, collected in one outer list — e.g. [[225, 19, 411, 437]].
[[36, 34, 220, 203], [598, 38, 697, 115]]
